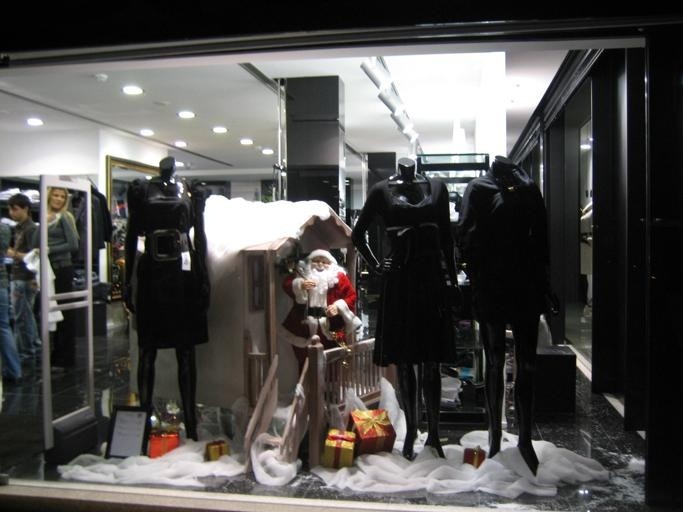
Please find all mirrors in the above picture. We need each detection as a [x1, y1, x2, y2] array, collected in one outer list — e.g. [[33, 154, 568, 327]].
[[106, 154, 161, 302]]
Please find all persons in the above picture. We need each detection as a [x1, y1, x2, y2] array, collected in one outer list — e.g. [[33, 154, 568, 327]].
[[0, 221, 23, 388], [119, 154, 214, 454], [33, 186, 80, 376], [351, 155, 461, 461], [6, 192, 42, 362], [455, 153, 571, 478]]
